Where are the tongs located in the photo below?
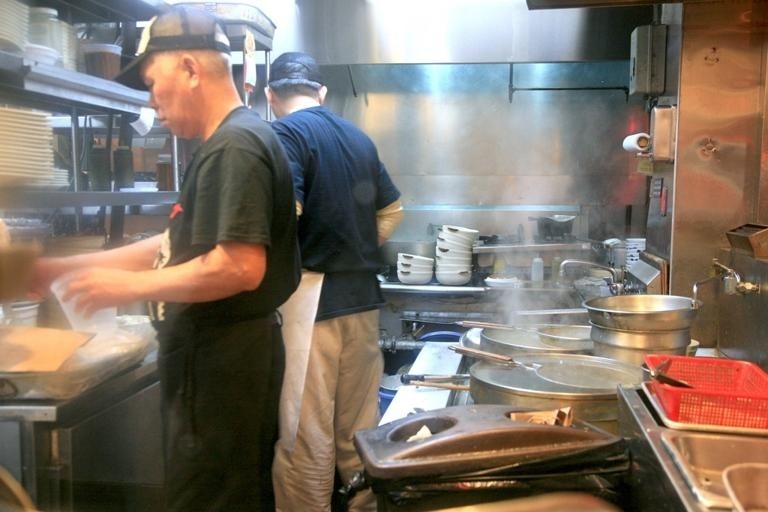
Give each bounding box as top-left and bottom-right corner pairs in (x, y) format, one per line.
(651, 358), (704, 388)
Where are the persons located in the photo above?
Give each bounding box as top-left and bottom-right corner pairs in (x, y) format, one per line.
(23, 7), (299, 512)
(263, 51), (405, 512)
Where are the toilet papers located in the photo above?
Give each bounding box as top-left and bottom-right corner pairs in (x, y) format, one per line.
(621, 132), (650, 153)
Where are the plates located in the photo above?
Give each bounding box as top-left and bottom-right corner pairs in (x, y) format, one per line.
(0, 0), (81, 71)
(0, 106), (72, 191)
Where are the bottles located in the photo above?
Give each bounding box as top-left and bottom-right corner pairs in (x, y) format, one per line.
(88, 143), (178, 193)
(531, 251), (563, 281)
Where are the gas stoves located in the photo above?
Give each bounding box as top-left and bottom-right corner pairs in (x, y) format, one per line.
(474, 232), (592, 252)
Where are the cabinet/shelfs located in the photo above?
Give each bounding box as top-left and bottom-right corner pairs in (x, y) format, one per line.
(0, 51), (192, 407)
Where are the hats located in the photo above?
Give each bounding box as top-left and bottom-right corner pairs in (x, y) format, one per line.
(267, 52), (322, 85)
(114, 9), (231, 91)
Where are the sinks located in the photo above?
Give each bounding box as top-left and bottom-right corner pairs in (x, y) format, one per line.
(507, 307), (591, 326)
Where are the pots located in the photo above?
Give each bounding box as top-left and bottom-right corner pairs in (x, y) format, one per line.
(530, 215), (577, 239)
(398, 309), (650, 436)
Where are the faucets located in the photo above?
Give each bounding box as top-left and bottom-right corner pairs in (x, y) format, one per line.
(691, 269), (725, 307)
(558, 259), (631, 297)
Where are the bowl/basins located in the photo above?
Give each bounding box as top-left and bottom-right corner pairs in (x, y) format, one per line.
(624, 236), (646, 272)
(394, 225), (480, 285)
(585, 293), (703, 332)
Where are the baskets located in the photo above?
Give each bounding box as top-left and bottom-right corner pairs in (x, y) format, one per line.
(644, 353), (768, 429)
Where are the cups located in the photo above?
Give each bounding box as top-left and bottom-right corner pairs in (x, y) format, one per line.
(48, 267), (117, 337)
(80, 42), (123, 82)
